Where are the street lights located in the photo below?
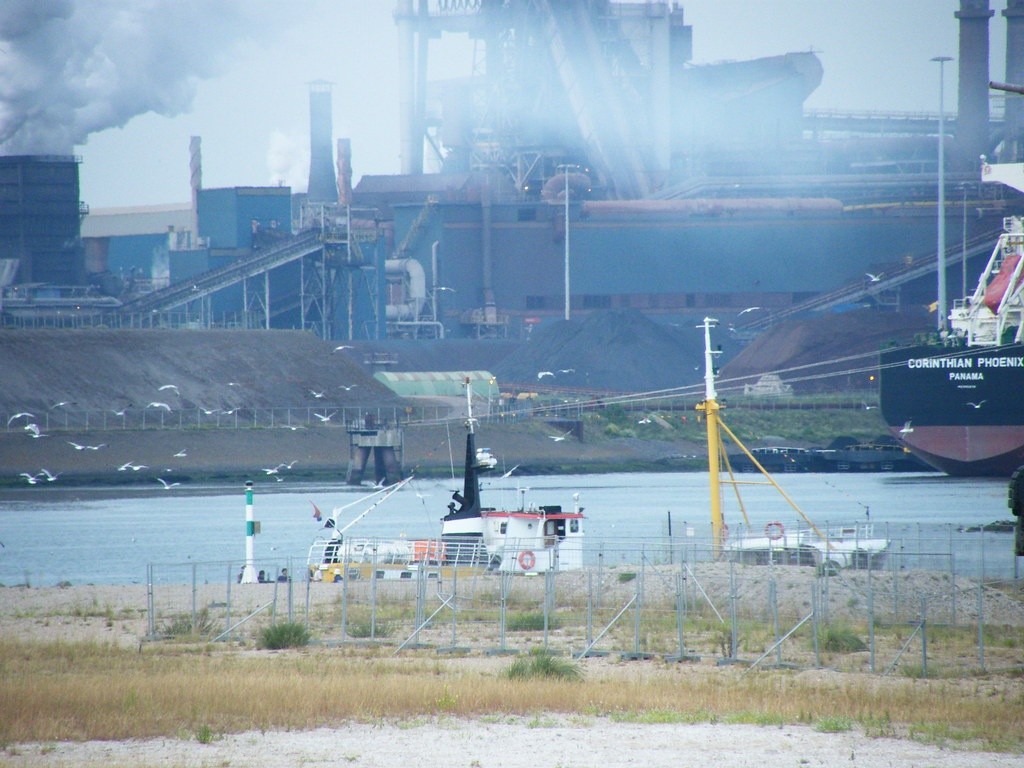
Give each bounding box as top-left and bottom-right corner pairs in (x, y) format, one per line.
(924, 55), (954, 331)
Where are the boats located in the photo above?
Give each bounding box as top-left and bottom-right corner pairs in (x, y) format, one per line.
(298, 373), (586, 577)
(878, 156), (1024, 483)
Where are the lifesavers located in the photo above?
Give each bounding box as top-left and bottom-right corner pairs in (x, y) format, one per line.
(821, 560), (841, 576)
(764, 520), (785, 541)
(851, 547), (868, 565)
(517, 550), (536, 570)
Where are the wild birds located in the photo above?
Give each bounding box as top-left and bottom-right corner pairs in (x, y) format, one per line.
(6, 273), (994, 500)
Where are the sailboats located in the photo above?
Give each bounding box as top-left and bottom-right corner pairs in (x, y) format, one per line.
(689, 314), (895, 572)
